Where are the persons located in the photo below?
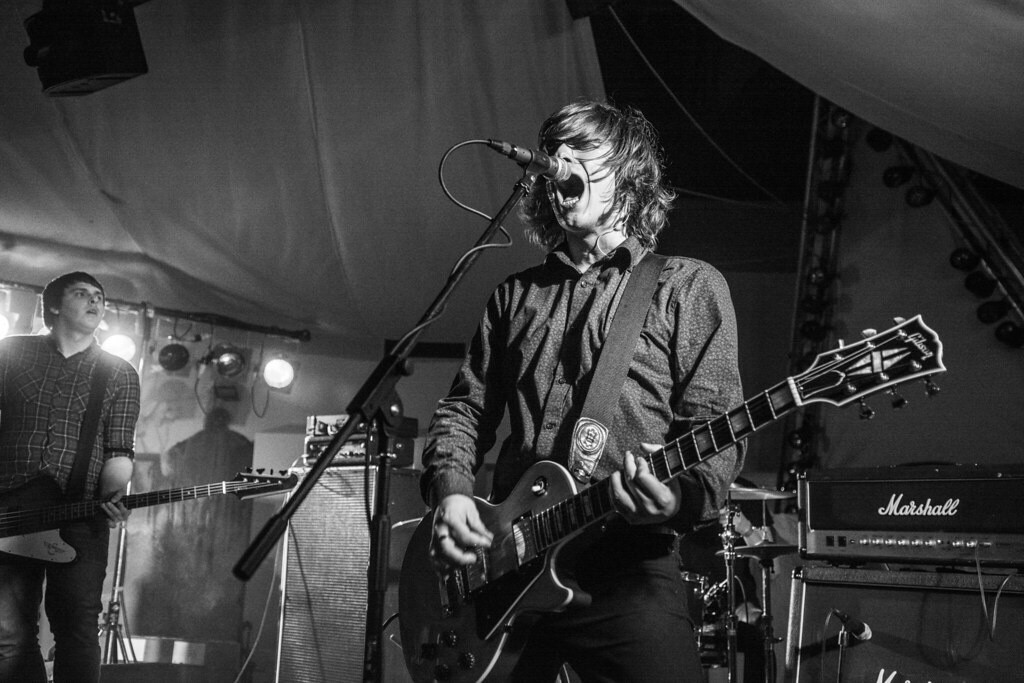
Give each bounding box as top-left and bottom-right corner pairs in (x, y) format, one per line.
(0, 269), (141, 683)
(418, 98), (749, 683)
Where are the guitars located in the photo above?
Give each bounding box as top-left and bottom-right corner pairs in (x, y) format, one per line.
(0, 465), (300, 566)
(400, 312), (949, 683)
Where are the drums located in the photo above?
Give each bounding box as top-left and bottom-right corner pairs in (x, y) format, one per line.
(676, 566), (730, 668)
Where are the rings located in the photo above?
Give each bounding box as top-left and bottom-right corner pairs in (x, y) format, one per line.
(435, 534), (451, 541)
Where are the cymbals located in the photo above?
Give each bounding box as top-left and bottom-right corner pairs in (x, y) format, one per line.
(714, 538), (810, 558)
(724, 479), (800, 501)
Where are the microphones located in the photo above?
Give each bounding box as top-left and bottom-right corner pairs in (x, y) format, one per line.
(834, 607), (872, 640)
(486, 138), (572, 184)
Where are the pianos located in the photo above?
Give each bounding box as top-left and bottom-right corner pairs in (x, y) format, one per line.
(797, 464), (1024, 566)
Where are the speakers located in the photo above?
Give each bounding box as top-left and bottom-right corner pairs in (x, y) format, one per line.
(271, 464), (431, 683)
(782, 565), (1024, 683)
